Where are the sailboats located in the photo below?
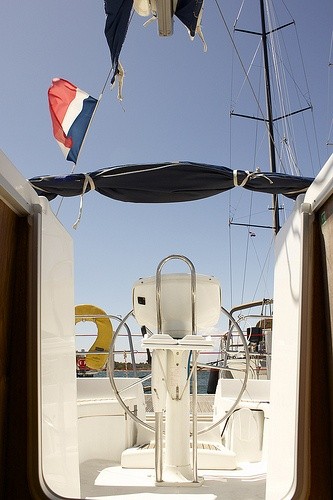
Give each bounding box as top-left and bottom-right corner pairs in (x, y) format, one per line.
(217, 1), (317, 380)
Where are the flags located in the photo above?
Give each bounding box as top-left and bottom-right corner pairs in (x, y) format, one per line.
(47, 76), (97, 167)
